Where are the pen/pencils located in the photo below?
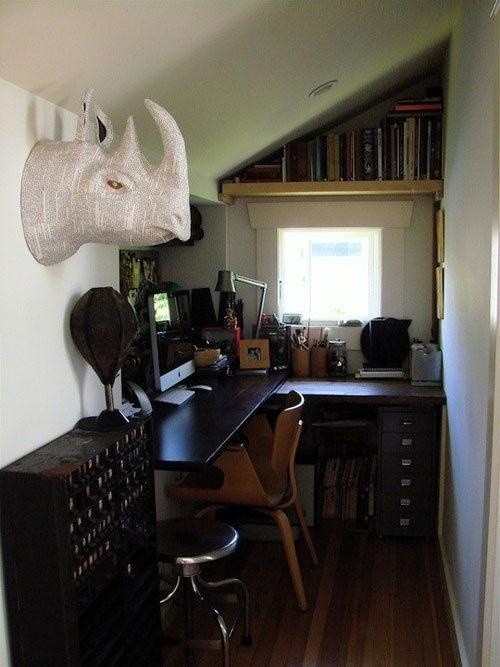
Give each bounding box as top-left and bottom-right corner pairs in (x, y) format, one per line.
(289, 327), (309, 350)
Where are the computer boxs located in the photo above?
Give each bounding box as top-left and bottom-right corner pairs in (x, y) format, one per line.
(221, 484), (301, 542)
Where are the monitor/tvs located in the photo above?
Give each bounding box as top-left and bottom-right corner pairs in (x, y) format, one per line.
(148, 290), (197, 392)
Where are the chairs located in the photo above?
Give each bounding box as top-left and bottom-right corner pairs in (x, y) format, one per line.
(165, 390), (319, 611)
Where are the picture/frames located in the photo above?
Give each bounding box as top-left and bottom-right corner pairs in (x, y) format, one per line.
(239, 340), (270, 369)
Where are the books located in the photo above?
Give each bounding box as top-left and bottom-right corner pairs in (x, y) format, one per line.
(352, 363), (405, 379)
(244, 95), (442, 183)
(322, 454), (377, 520)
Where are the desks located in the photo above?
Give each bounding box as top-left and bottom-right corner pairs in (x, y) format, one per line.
(146, 364), (285, 470)
(267, 374), (445, 404)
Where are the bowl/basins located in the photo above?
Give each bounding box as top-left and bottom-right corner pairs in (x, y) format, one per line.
(194, 349), (219, 366)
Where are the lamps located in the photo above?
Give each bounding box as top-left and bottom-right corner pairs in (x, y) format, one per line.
(213, 271), (267, 339)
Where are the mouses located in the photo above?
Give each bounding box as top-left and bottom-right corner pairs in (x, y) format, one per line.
(191, 385), (212, 391)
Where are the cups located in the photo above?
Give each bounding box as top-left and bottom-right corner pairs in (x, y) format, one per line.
(290, 348), (310, 377)
(310, 346), (328, 377)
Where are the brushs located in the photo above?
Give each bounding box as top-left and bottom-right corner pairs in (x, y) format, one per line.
(323, 328), (330, 343)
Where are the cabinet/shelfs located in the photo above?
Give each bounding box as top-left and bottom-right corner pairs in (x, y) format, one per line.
(374, 406), (438, 540)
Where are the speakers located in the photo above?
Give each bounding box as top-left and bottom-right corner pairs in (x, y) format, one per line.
(125, 379), (154, 415)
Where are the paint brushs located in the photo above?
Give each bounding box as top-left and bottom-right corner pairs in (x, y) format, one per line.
(325, 326), (328, 342)
(320, 327), (323, 342)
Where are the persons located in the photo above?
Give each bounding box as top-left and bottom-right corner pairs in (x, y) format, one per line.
(248, 348), (261, 360)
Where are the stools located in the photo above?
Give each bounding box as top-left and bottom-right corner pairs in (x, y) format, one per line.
(156, 517), (252, 667)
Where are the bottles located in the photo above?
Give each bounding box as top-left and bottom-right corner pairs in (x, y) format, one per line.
(326, 341), (347, 378)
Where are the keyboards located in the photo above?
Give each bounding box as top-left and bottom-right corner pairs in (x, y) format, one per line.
(152, 387), (195, 407)
